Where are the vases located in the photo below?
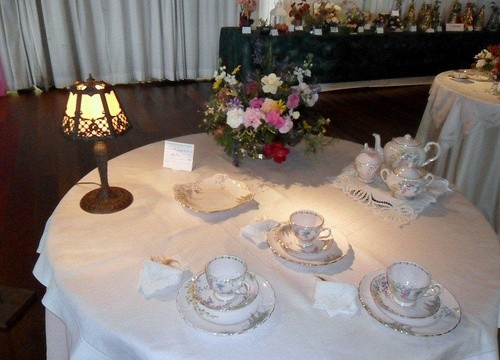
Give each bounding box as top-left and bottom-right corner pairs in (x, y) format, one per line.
(240, 22), (250, 31)
(356, 24), (363, 33)
(493, 71), (500, 81)
(321, 20), (329, 33)
(249, 129), (285, 159)
(294, 20), (303, 31)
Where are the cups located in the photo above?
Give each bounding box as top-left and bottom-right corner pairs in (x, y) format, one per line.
(205, 254), (250, 296)
(386, 261), (443, 309)
(289, 209), (332, 247)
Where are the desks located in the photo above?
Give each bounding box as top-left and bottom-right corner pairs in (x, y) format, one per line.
(35, 128), (500, 360)
(413, 68), (500, 249)
(220, 26), (498, 84)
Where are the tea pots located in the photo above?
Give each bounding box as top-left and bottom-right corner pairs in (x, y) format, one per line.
(371, 133), (442, 171)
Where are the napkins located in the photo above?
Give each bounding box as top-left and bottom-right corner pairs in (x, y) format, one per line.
(313, 277), (362, 321)
(240, 218), (283, 247)
(136, 255), (189, 297)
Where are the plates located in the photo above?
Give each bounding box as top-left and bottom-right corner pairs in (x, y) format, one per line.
(370, 273), (441, 319)
(266, 221), (350, 266)
(273, 220), (335, 255)
(357, 269), (462, 338)
(177, 271), (276, 336)
(171, 172), (254, 215)
(191, 270), (259, 310)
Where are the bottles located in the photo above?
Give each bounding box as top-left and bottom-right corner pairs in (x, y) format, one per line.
(379, 161), (434, 200)
(354, 143), (380, 183)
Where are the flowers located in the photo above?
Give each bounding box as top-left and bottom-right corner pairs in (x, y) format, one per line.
(472, 44), (500, 70)
(375, 12), (402, 26)
(312, 1), (340, 20)
(344, 8), (372, 26)
(237, 0), (258, 26)
(193, 51), (331, 164)
(287, 1), (312, 20)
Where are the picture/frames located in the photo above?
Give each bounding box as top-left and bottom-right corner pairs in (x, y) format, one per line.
(383, 26), (390, 32)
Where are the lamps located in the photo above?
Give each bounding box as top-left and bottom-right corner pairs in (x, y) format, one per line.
(61, 74), (137, 216)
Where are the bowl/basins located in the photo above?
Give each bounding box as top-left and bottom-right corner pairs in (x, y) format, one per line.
(454, 70), (467, 79)
